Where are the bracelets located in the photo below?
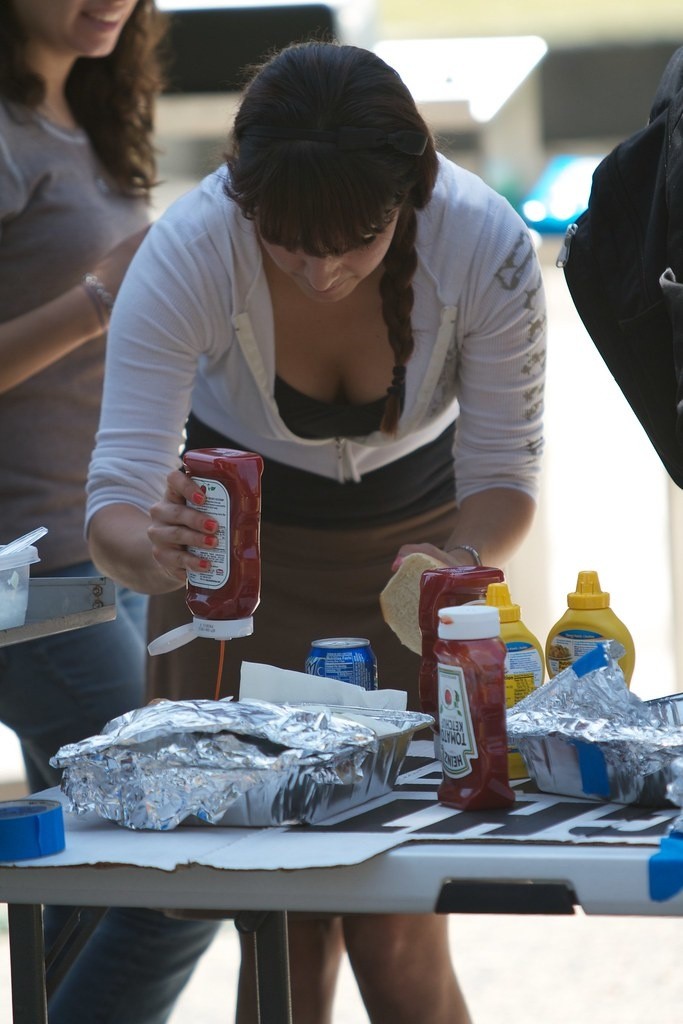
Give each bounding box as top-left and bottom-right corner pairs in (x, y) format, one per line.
(81, 272), (115, 331)
(446, 542), (483, 566)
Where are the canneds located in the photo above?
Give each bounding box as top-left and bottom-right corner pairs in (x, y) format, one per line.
(304, 637), (378, 691)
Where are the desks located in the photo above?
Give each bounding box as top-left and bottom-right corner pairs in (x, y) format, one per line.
(0, 739), (683, 1024)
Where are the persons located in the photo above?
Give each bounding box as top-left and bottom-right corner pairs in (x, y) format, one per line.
(81, 42), (550, 1024)
(0, 0), (225, 1024)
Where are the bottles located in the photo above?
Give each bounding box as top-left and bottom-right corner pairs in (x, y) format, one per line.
(473, 582), (546, 779)
(435, 605), (515, 810)
(545, 570), (635, 693)
(418, 564), (505, 764)
(178, 446), (263, 640)
